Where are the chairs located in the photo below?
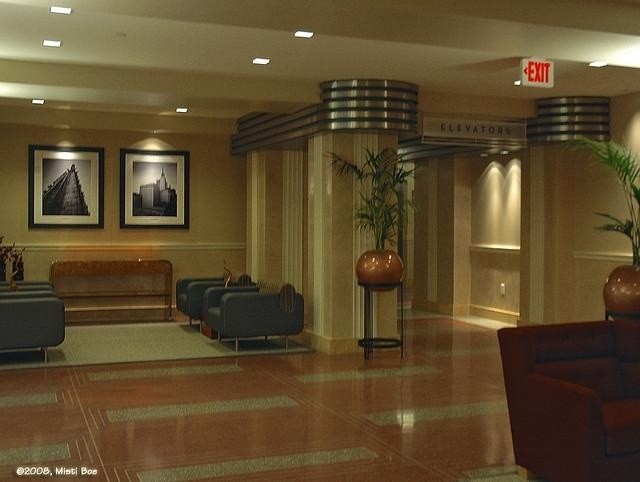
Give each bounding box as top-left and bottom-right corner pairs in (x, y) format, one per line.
(0, 279), (54, 291)
(202, 279), (305, 352)
(0, 290), (66, 362)
(176, 268), (251, 330)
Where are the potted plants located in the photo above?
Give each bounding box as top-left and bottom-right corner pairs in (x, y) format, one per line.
(325, 146), (418, 288)
(568, 132), (639, 319)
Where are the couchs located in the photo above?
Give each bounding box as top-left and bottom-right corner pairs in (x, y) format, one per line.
(497, 320), (640, 482)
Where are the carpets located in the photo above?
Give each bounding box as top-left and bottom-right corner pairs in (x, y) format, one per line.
(0, 319), (316, 371)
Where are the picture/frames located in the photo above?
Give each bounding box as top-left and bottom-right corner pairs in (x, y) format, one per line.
(120, 148), (192, 230)
(27, 144), (105, 230)
